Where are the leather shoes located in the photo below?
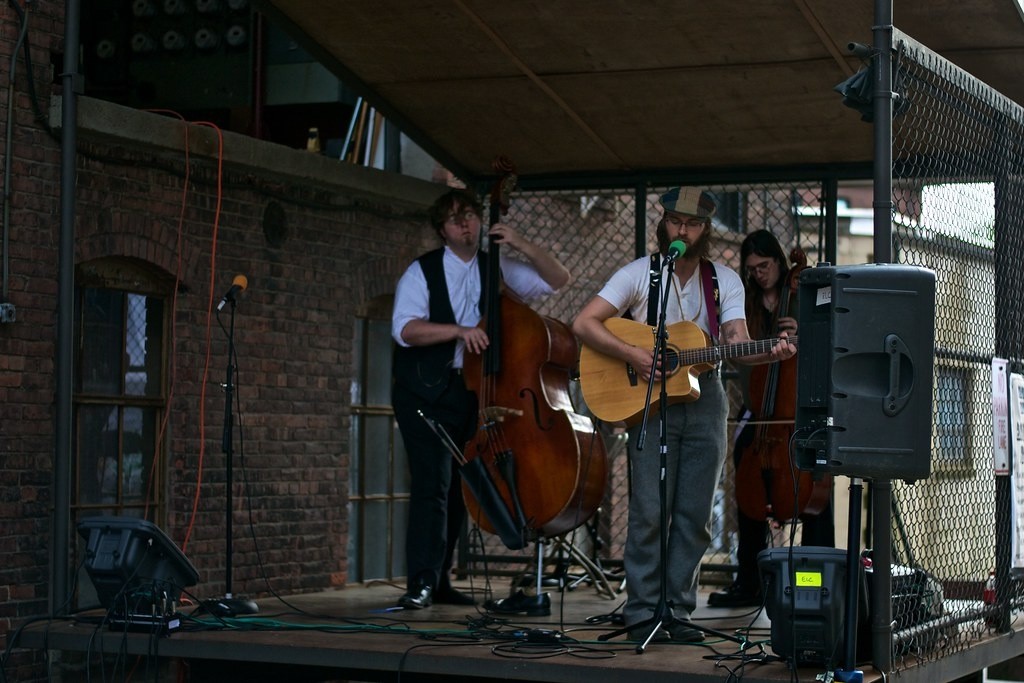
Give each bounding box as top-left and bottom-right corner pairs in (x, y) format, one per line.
(398, 584), (432, 610)
(483, 589), (550, 617)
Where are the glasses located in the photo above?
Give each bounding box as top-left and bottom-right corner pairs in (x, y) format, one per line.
(746, 263), (775, 274)
(665, 213), (706, 231)
(445, 210), (479, 225)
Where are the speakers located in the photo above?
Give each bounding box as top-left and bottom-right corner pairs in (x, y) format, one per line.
(793, 262), (935, 484)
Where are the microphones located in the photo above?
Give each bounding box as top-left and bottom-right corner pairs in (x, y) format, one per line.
(217, 275), (248, 312)
(662, 240), (686, 266)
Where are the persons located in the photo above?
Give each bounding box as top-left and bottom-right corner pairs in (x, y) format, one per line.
(706, 230), (838, 610)
(390, 190), (570, 610)
(573, 187), (797, 644)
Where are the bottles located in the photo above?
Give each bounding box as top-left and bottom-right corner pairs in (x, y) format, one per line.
(984, 572), (997, 627)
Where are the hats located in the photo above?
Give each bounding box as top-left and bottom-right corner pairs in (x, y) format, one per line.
(659, 185), (717, 222)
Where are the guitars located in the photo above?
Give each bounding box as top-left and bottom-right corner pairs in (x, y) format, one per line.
(577, 313), (798, 430)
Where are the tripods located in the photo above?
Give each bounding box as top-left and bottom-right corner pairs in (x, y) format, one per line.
(598, 255), (750, 653)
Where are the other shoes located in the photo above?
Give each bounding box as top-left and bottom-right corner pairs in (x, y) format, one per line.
(665, 619), (706, 642)
(626, 622), (670, 642)
(709, 586), (762, 608)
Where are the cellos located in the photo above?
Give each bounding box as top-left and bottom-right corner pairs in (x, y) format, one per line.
(733, 244), (834, 522)
(458, 153), (613, 597)
